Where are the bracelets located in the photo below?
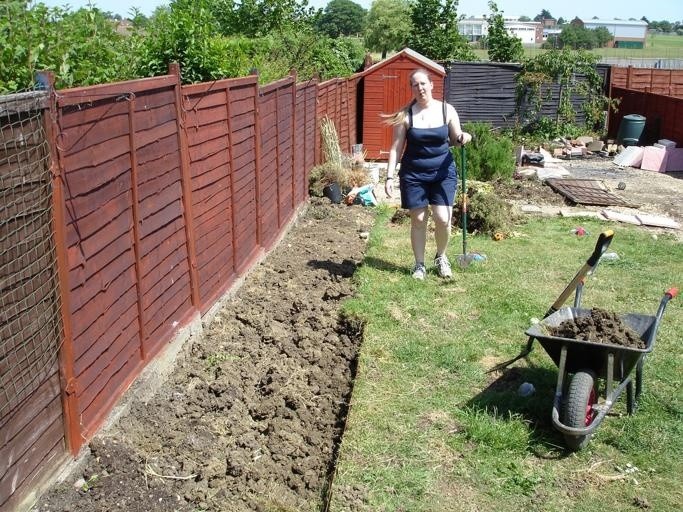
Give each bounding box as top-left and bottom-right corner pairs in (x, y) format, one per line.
(387, 177), (394, 181)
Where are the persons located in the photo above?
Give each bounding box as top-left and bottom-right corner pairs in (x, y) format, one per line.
(384, 68), (472, 280)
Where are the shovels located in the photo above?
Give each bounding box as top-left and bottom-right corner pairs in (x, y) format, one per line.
(454, 139), (486, 267)
(486, 229), (615, 374)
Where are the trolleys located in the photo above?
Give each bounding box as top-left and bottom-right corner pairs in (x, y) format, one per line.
(524, 252), (680, 451)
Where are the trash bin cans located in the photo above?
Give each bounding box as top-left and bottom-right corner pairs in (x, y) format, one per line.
(617, 114), (647, 145)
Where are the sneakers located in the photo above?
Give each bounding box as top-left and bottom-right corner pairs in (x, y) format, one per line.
(433, 251), (453, 278)
(410, 264), (427, 282)
